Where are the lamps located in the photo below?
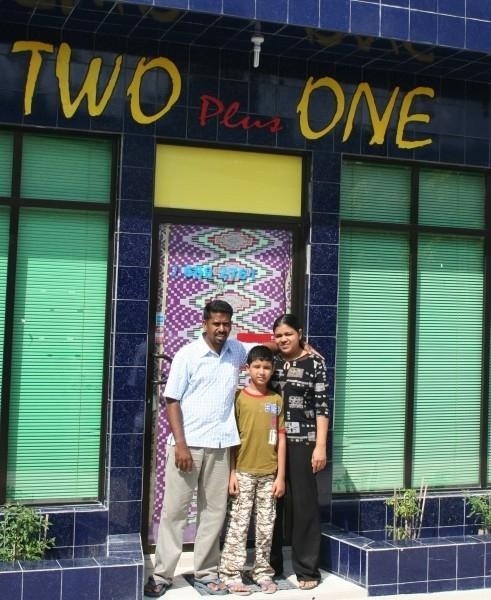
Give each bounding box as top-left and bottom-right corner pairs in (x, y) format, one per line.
(250, 32), (264, 70)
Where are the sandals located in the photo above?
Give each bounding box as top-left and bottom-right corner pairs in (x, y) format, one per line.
(194, 578), (228, 595)
(299, 579), (320, 590)
(254, 578), (277, 594)
(145, 578), (174, 599)
(223, 581), (252, 597)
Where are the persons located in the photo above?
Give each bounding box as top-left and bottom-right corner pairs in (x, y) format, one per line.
(268, 315), (330, 590)
(218, 347), (286, 596)
(144, 300), (326, 596)
